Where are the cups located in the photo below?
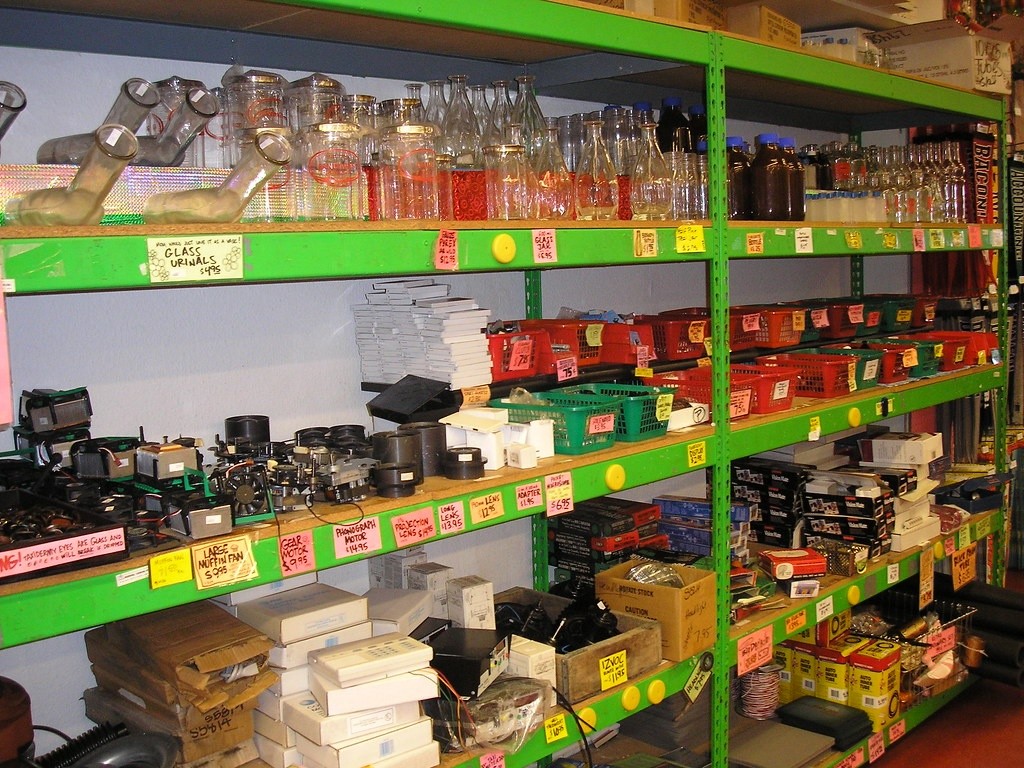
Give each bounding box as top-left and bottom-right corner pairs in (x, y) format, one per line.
(158, 67), (452, 225)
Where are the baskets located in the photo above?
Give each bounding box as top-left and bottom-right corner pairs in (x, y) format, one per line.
(476, 291), (944, 382)
(486, 392), (626, 456)
(633, 328), (1001, 421)
(549, 384), (678, 443)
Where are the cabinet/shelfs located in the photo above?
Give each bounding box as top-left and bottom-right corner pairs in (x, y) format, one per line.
(0, 0), (1024, 768)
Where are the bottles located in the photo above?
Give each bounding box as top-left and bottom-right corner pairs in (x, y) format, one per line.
(803, 38), (855, 60)
(751, 133), (805, 220)
(883, 142), (968, 223)
(726, 136), (751, 220)
(405, 74), (708, 221)
(804, 141), (883, 192)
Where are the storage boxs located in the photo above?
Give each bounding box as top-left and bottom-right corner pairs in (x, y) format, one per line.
(585, 0), (1024, 96)
(79, 375), (1002, 768)
